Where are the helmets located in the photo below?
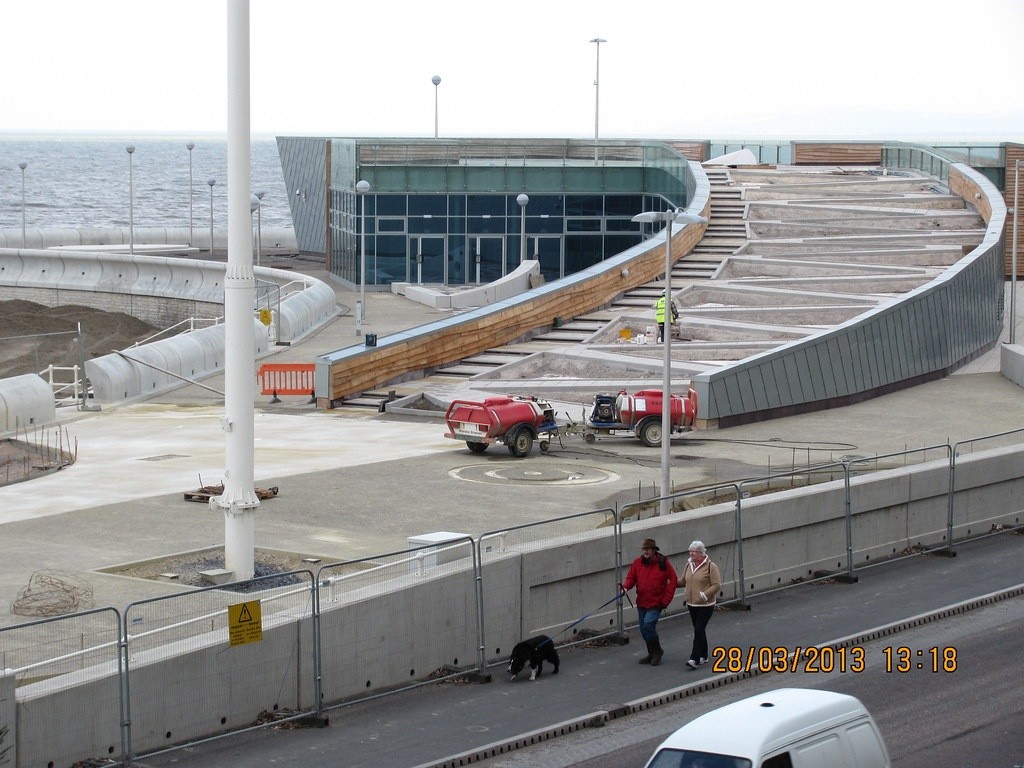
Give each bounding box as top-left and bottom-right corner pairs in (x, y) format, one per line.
(662, 288), (666, 296)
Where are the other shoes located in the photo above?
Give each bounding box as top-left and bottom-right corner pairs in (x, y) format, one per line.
(640, 655), (652, 664)
(651, 650), (663, 665)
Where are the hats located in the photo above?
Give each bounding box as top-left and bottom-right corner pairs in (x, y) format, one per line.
(640, 539), (660, 551)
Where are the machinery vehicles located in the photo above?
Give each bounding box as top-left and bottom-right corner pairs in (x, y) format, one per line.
(443, 393), (559, 458)
(587, 388), (697, 447)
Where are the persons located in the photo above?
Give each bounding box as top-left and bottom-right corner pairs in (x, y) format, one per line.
(620, 539), (677, 666)
(668, 541), (721, 669)
(656, 289), (679, 342)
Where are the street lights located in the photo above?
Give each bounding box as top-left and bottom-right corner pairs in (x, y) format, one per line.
(589, 38), (608, 165)
(19, 161), (28, 246)
(254, 190), (264, 267)
(126, 143), (135, 254)
(631, 210), (704, 516)
(356, 179), (370, 320)
(208, 178), (215, 254)
(432, 75), (441, 137)
(186, 142), (194, 246)
(517, 193), (529, 264)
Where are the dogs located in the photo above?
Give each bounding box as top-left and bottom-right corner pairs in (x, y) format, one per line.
(507, 634), (560, 681)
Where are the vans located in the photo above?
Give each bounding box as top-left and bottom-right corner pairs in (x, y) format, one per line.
(644, 688), (892, 768)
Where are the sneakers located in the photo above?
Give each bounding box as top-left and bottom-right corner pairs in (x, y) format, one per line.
(699, 657), (709, 664)
(686, 660), (698, 668)
